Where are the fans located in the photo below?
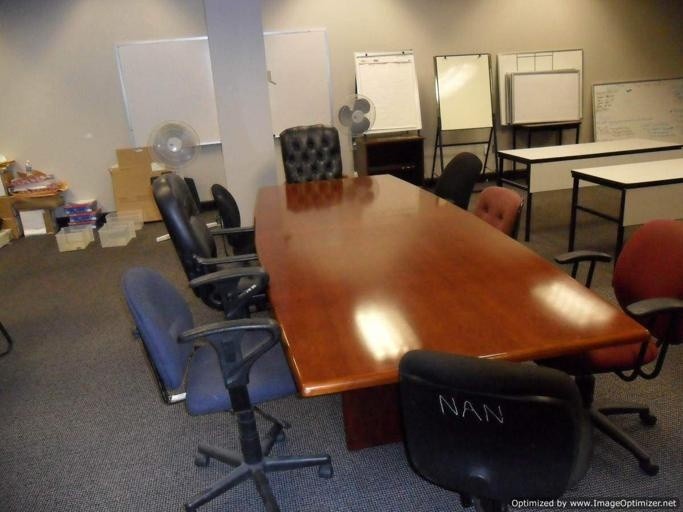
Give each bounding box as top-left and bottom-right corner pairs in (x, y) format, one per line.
(147, 120), (222, 242)
(335, 93), (376, 177)
(345, 177), (378, 208)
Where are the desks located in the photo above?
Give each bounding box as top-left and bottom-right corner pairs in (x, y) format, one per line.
(513, 121), (582, 179)
(498, 137), (682, 242)
(566, 157), (683, 287)
(256, 174), (650, 453)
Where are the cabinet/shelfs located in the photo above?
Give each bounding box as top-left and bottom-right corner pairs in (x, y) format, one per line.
(356, 135), (427, 189)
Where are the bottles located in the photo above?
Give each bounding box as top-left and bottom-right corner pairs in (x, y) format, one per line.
(25, 160), (32, 176)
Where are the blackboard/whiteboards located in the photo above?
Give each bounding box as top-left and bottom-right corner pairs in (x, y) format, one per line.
(592, 77), (683, 142)
(354, 51), (421, 137)
(495, 49), (584, 127)
(433, 53), (492, 131)
(511, 71), (581, 123)
(115, 29), (335, 151)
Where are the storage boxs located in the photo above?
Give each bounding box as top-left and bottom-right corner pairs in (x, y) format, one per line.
(54, 200), (102, 253)
(0, 195), (65, 249)
(97, 145), (166, 248)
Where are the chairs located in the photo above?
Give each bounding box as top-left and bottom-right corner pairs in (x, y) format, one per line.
(285, 180), (343, 211)
(399, 349), (588, 512)
(121, 265), (334, 512)
(435, 152), (483, 210)
(279, 124), (343, 182)
(534, 219), (683, 475)
(153, 173), (271, 317)
(473, 186), (525, 241)
